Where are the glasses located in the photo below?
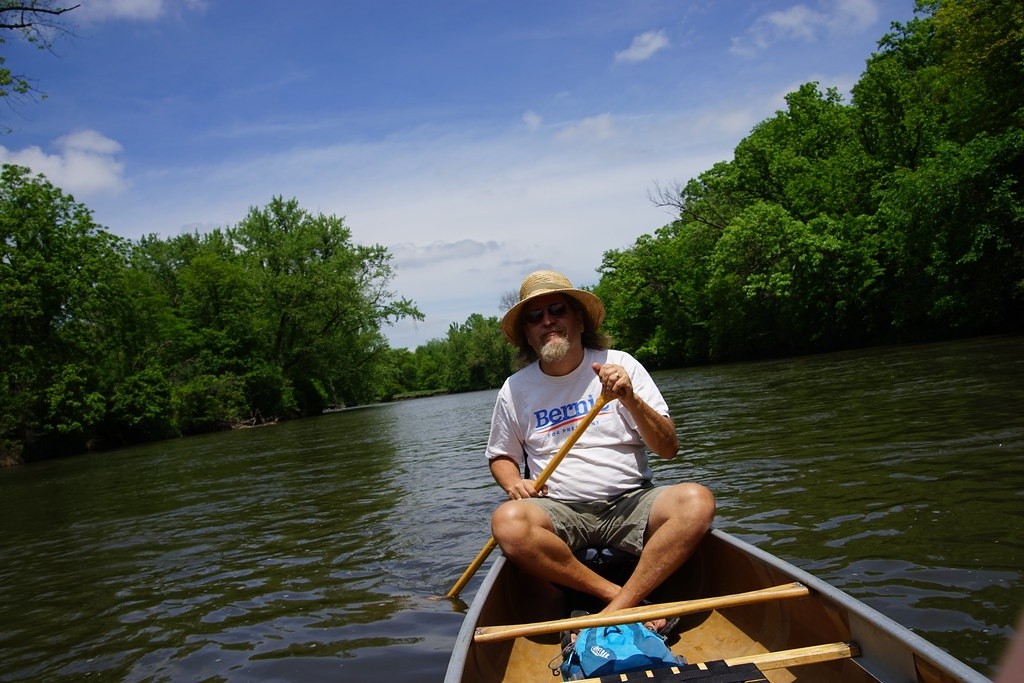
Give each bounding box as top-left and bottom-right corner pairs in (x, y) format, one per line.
(522, 303), (572, 325)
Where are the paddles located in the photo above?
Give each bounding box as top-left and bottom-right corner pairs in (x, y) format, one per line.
(446, 393), (618, 598)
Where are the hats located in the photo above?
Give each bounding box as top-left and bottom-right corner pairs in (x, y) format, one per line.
(501, 270), (605, 348)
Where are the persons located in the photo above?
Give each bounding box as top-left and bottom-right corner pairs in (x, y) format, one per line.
(485, 270), (715, 657)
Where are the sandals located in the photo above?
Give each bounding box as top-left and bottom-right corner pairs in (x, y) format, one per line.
(643, 616), (680, 640)
(560, 610), (590, 660)
